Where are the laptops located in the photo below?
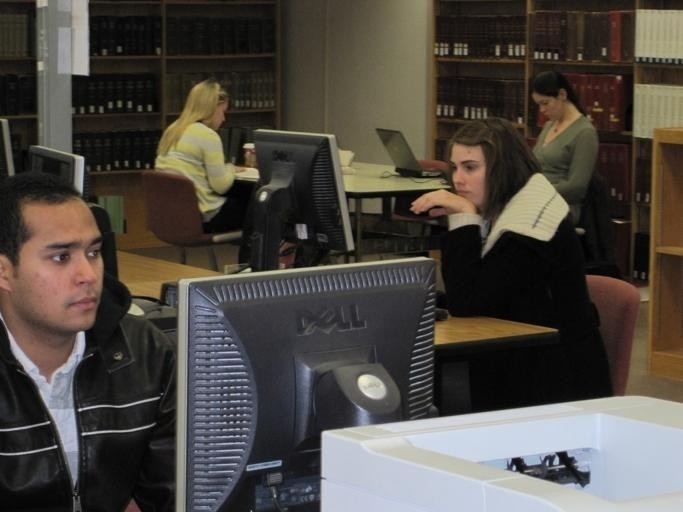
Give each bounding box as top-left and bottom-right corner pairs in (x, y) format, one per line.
(375, 128), (444, 178)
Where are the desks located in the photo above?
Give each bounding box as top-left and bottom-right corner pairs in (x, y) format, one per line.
(117, 251), (563, 416)
(231, 160), (451, 260)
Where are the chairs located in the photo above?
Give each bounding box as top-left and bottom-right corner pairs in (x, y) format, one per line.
(584, 273), (639, 395)
(142, 170), (246, 271)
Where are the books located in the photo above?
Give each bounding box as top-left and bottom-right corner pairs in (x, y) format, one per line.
(1, 1), (277, 60)
(434, 1), (680, 284)
(0, 71), (276, 172)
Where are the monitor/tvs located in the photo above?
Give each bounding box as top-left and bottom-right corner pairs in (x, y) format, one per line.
(27, 144), (90, 203)
(0, 118), (16, 178)
(174, 255), (437, 511)
(253, 128), (355, 253)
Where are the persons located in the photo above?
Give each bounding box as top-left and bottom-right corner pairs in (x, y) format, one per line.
(152, 76), (257, 268)
(410, 118), (614, 414)
(0, 171), (176, 512)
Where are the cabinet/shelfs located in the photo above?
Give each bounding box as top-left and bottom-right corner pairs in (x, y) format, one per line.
(423, 0), (683, 383)
(0, 0), (284, 249)
